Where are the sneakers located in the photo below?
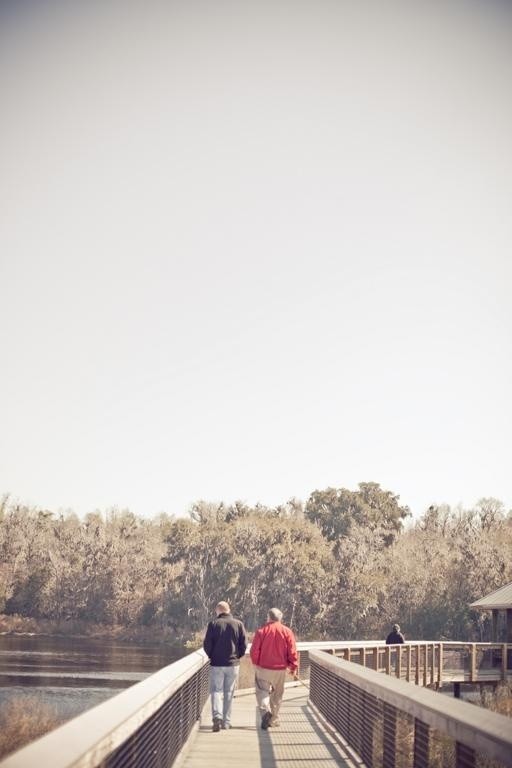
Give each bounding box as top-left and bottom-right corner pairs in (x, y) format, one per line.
(212, 711), (280, 732)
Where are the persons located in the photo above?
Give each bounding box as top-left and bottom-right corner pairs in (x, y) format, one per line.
(250, 606), (300, 730)
(386, 623), (405, 644)
(203, 600), (248, 732)
